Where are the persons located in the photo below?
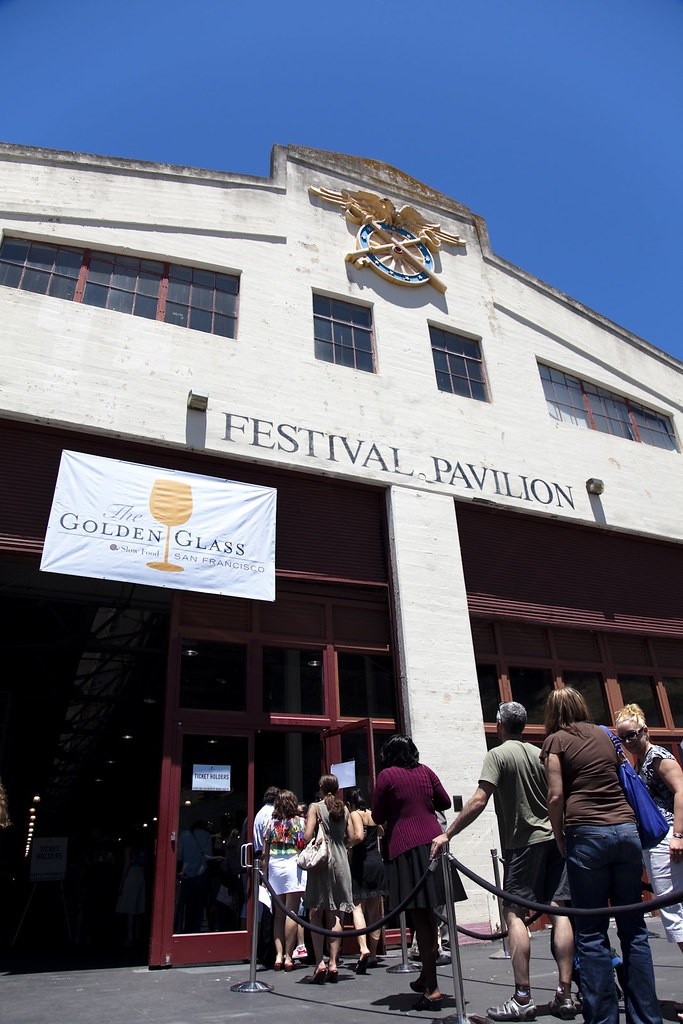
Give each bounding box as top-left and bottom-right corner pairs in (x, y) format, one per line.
(253, 774), (451, 982)
(371, 734), (451, 1011)
(430, 687), (683, 1024)
(114, 801), (247, 945)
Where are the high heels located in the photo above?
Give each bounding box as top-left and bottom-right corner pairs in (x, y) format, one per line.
(327, 969), (338, 983)
(412, 994), (441, 1011)
(309, 967), (327, 985)
(410, 975), (427, 993)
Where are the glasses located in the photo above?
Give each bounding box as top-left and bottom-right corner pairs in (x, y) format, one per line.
(618, 726), (644, 742)
(497, 701), (508, 714)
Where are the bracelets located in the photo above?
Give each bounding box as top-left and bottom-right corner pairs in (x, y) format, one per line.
(673, 833), (683, 838)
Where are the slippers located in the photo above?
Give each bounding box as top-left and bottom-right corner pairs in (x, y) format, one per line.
(356, 953), (372, 975)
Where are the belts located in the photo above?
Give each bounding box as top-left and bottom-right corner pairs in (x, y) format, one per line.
(255, 850), (262, 857)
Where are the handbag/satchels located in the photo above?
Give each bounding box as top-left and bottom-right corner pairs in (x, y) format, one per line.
(600, 725), (670, 850)
(196, 867), (214, 897)
(296, 803), (329, 871)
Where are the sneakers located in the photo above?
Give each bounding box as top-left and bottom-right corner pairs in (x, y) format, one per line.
(548, 994), (576, 1016)
(486, 995), (537, 1021)
(283, 947), (308, 958)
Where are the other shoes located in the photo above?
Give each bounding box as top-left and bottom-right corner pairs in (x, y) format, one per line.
(327, 958), (339, 966)
(436, 955), (452, 965)
(410, 954), (422, 961)
(284, 963), (295, 972)
(274, 962), (284, 971)
(366, 956), (377, 968)
